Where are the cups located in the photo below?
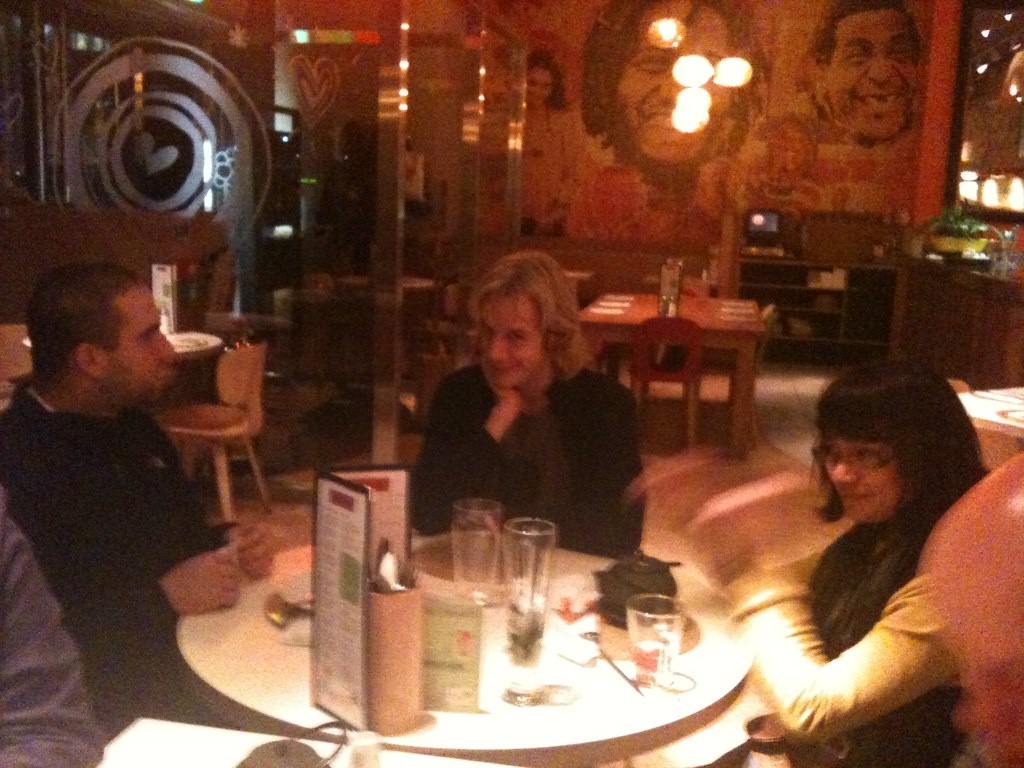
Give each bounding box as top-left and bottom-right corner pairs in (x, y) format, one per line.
(625, 593), (686, 686)
(450, 498), (505, 604)
(503, 517), (558, 631)
(366, 587), (425, 733)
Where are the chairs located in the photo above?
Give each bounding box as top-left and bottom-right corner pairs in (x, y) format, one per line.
(630, 316), (705, 446)
(157, 342), (273, 526)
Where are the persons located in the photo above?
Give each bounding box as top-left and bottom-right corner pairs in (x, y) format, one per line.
(0, 258), (282, 768)
(405, 251), (646, 560)
(695, 365), (1024, 768)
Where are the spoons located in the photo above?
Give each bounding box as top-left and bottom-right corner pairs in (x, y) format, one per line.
(582, 631), (643, 695)
(377, 550), (399, 593)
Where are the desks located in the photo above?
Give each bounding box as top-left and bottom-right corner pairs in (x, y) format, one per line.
(578, 291), (767, 460)
(175, 533), (754, 768)
(98, 717), (522, 768)
(164, 332), (225, 360)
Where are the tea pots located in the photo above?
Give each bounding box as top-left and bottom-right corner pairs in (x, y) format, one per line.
(595, 549), (683, 613)
(234, 721), (346, 768)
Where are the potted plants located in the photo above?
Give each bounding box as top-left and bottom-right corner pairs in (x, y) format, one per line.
(987, 225), (1018, 279)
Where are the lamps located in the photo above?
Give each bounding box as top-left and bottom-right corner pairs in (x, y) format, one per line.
(648, 0), (754, 134)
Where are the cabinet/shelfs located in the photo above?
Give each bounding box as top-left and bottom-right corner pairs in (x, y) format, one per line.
(732, 256), (895, 348)
(927, 198), (990, 253)
